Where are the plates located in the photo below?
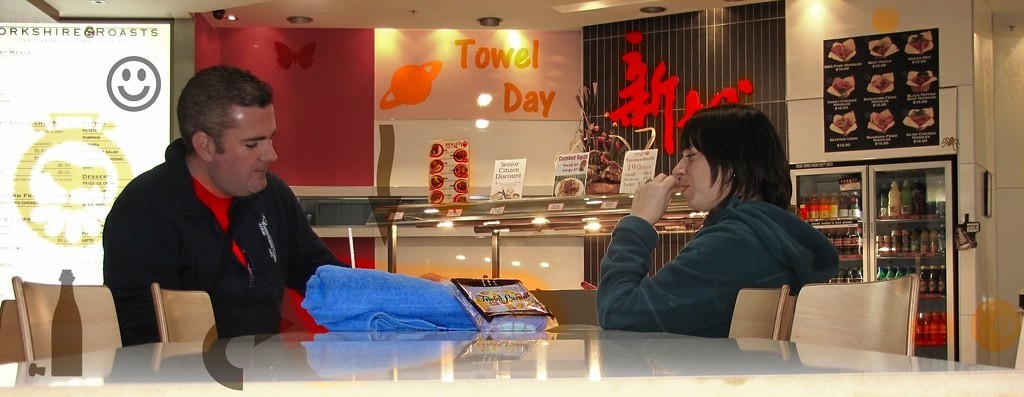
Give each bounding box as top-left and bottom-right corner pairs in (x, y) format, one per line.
(554, 178), (585, 198)
(454, 179), (468, 194)
(430, 159), (444, 173)
(453, 149), (468, 163)
(429, 144), (444, 157)
(453, 193), (466, 203)
(430, 175), (444, 190)
(429, 189), (444, 204)
(453, 164), (469, 178)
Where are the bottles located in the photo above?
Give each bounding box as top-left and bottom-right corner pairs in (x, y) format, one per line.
(798, 175), (948, 346)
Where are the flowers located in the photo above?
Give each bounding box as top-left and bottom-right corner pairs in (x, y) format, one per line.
(567, 83), (626, 183)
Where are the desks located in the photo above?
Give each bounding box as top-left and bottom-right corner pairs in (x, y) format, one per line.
(1, 326), (1015, 389)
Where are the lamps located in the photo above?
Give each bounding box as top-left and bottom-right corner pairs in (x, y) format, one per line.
(479, 18), (500, 27)
(290, 17), (308, 24)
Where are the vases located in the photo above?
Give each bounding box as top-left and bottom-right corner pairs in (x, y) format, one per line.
(587, 183), (619, 195)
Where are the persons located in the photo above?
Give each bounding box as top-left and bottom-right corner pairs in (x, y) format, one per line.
(102, 65), (352, 347)
(595, 103), (839, 338)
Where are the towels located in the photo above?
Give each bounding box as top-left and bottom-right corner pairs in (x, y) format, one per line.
(301, 264), (480, 333)
(297, 331), (469, 380)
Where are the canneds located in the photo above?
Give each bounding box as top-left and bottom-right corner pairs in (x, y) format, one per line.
(875, 228), (910, 257)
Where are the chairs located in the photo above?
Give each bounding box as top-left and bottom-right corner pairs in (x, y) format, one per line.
(729, 282), (790, 341)
(788, 272), (921, 357)
(0, 299), (25, 366)
(152, 282), (218, 343)
(11, 276), (123, 363)
